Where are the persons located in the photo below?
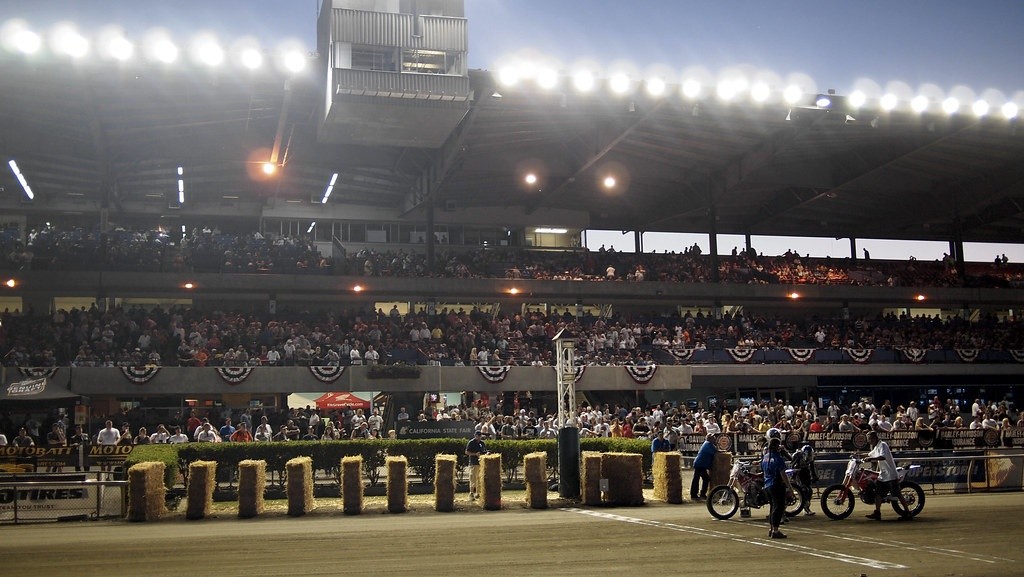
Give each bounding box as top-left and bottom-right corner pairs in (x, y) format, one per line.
(825, 395), (1024, 454)
(0, 223), (336, 273)
(717, 247), (1024, 290)
(690, 433), (717, 504)
(389, 393), (558, 440)
(465, 430), (489, 501)
(562, 396), (825, 471)
(0, 302), (466, 367)
(753, 428), (822, 539)
(853, 430), (913, 521)
(0, 403), (273, 471)
(467, 306), (802, 367)
(273, 405), (384, 442)
(352, 233), (710, 283)
(806, 310), (1024, 350)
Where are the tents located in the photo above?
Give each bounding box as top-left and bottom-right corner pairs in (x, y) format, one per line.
(0, 376), (92, 446)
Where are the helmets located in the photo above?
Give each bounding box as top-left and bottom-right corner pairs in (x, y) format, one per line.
(802, 444), (814, 461)
(767, 427), (780, 438)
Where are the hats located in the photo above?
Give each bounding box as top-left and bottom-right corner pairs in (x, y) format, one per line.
(19, 427), (26, 432)
(122, 421), (130, 428)
(666, 417), (673, 421)
(708, 413), (715, 417)
(898, 414), (903, 417)
(706, 433), (715, 439)
(520, 409), (526, 413)
(474, 430), (481, 435)
(280, 424), (287, 429)
(909, 400), (917, 406)
(658, 430), (663, 435)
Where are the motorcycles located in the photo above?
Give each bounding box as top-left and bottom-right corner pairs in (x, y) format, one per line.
(706, 460), (806, 519)
(821, 453), (925, 519)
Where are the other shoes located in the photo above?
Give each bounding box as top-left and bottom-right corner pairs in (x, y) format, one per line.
(768, 528), (787, 539)
(897, 512), (913, 520)
(691, 495), (707, 500)
(806, 511), (815, 515)
(866, 511), (882, 521)
